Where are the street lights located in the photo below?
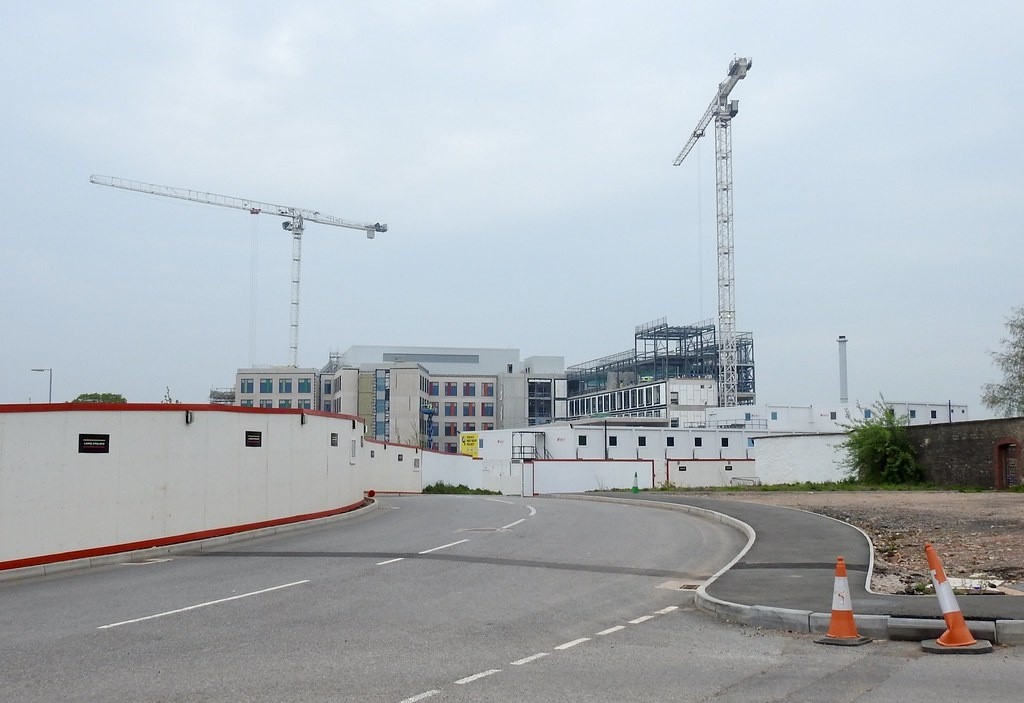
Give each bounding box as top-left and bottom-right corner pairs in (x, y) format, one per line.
(30, 368), (53, 404)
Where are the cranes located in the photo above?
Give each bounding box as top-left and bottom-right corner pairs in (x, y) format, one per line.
(671, 54), (756, 408)
(87, 174), (387, 366)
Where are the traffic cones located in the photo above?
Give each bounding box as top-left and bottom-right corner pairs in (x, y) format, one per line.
(812, 556), (874, 646)
(920, 543), (995, 654)
